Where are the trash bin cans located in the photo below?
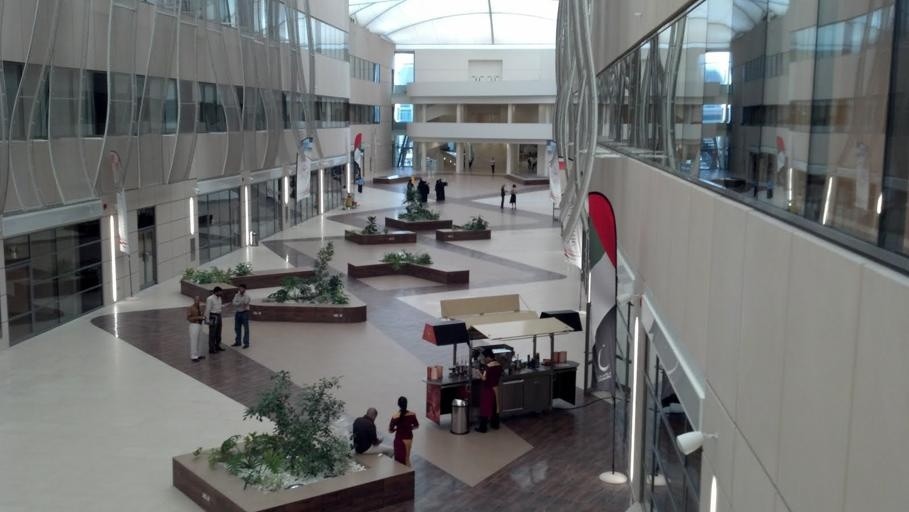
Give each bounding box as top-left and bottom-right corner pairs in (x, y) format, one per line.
(250, 231), (258, 246)
(451, 399), (469, 434)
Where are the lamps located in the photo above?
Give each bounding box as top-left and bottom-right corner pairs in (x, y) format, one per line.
(617, 293), (642, 306)
(676, 431), (719, 456)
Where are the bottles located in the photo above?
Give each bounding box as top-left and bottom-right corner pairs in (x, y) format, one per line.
(456, 361), (468, 377)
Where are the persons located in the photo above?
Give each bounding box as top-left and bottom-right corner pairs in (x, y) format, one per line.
(509, 184), (517, 211)
(231, 284), (251, 348)
(526, 151), (538, 172)
(204, 286), (225, 353)
(403, 175), (447, 207)
(489, 157), (496, 175)
(345, 193), (357, 209)
(500, 184), (508, 209)
(474, 349), (504, 433)
(357, 174), (362, 193)
(352, 408), (394, 459)
(388, 395), (420, 467)
(341, 185), (348, 210)
(185, 296), (205, 361)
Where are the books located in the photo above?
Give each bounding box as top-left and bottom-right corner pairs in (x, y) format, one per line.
(206, 315), (218, 324)
